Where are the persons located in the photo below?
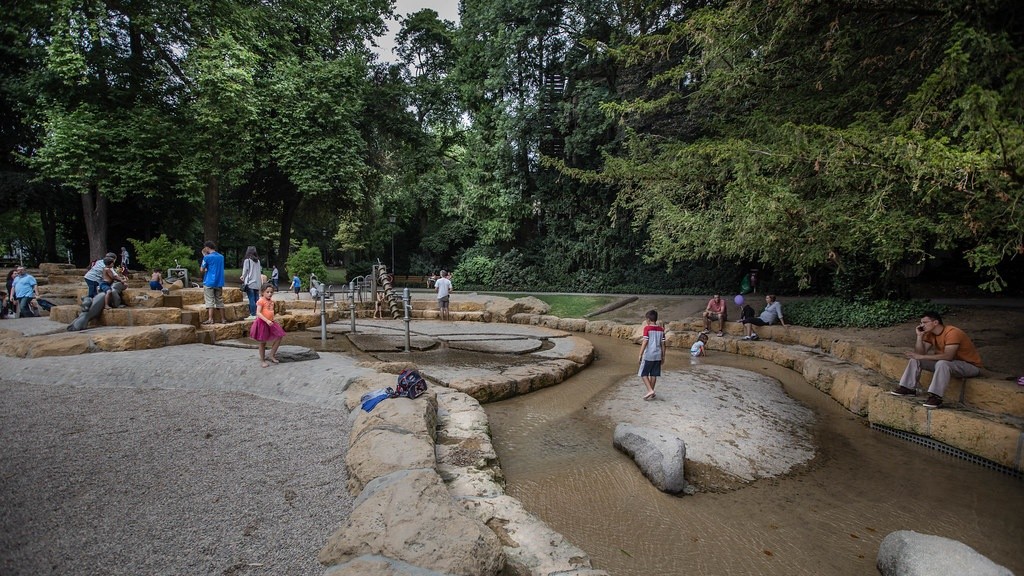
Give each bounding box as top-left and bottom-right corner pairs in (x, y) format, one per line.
(691, 333), (708, 357)
(239, 246), (268, 321)
(80, 252), (129, 310)
(427, 272), (453, 289)
(637, 310), (666, 401)
(120, 247), (129, 281)
(373, 291), (384, 319)
(250, 283), (286, 368)
(699, 293), (727, 337)
(735, 294), (791, 341)
(200, 240), (226, 324)
(149, 267), (163, 290)
(434, 270), (453, 321)
(289, 273), (301, 301)
(0, 266), (57, 319)
(890, 311), (983, 407)
(271, 265), (279, 292)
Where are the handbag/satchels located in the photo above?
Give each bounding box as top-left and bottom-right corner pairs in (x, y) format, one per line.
(240, 284), (249, 291)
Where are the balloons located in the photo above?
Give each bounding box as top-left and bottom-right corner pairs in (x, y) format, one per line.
(734, 295), (744, 306)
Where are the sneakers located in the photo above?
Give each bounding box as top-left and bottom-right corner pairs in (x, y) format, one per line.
(922, 396), (943, 408)
(890, 386), (916, 397)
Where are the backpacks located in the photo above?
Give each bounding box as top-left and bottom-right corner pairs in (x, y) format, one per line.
(395, 369), (427, 399)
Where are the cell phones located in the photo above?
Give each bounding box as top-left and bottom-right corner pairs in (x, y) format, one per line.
(919, 327), (924, 331)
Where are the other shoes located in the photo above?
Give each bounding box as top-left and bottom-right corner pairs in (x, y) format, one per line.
(702, 329), (710, 334)
(743, 335), (758, 340)
(219, 319), (226, 324)
(717, 331), (723, 337)
(244, 315), (257, 320)
(203, 319), (214, 324)
